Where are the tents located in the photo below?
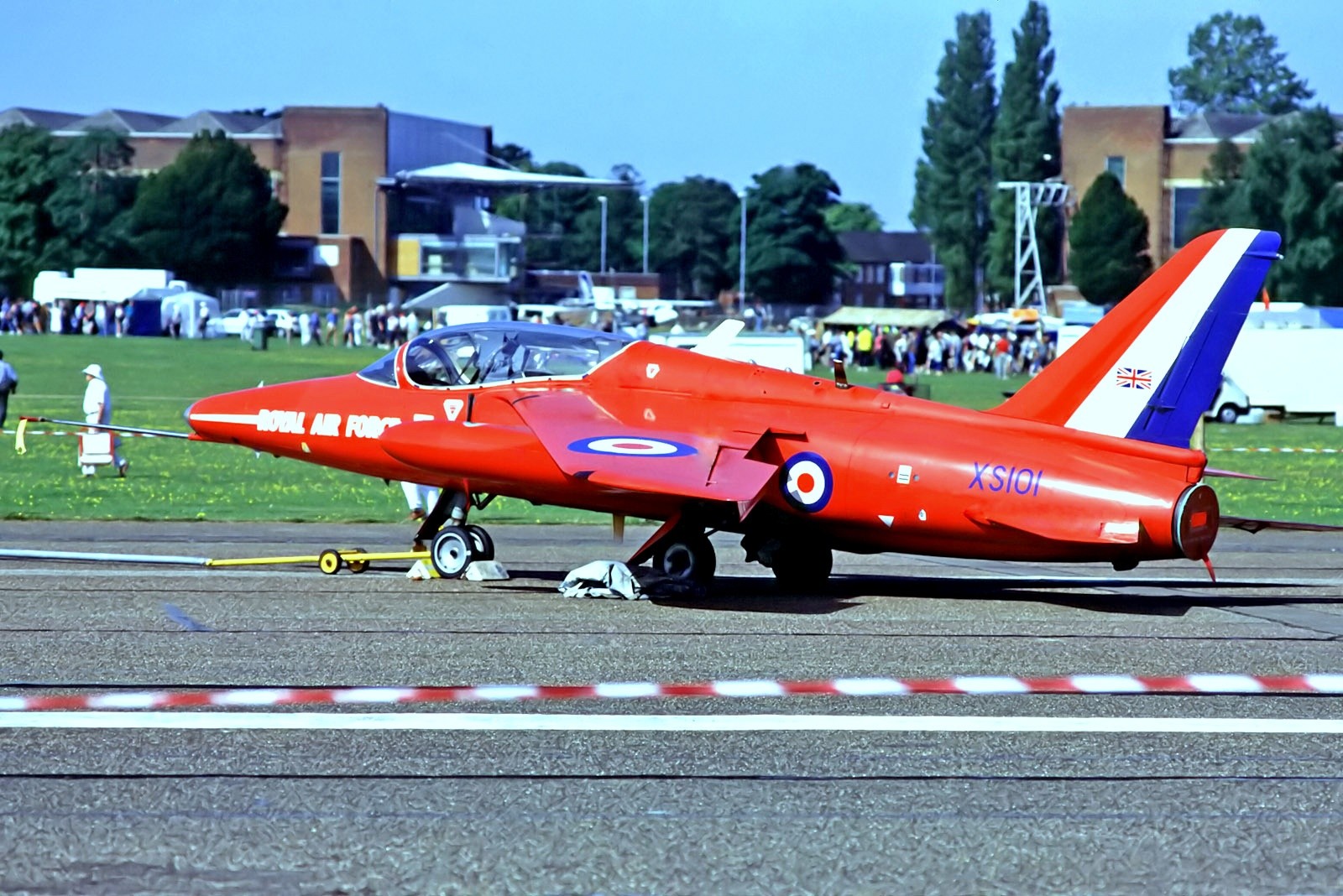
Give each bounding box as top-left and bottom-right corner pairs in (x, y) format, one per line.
(821, 306), (967, 330)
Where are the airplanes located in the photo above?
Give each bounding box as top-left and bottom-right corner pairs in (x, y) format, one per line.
(17, 225), (1286, 601)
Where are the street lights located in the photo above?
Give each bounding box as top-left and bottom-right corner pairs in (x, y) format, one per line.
(597, 195), (607, 270)
(638, 195), (651, 273)
(735, 191), (749, 316)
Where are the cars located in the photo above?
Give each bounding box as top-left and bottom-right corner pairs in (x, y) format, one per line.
(1205, 372), (1251, 426)
(264, 308), (301, 339)
(205, 307), (275, 337)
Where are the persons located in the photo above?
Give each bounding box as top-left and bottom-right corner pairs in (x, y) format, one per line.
(0, 349), (18, 429)
(636, 317), (650, 342)
(741, 296), (1057, 381)
(401, 481), (439, 522)
(537, 312), (564, 326)
(80, 364), (130, 479)
(612, 303), (627, 332)
(0, 280), (435, 353)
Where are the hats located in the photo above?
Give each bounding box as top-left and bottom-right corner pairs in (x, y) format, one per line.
(82, 364), (104, 380)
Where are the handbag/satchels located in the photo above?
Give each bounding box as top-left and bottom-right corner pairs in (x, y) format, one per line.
(79, 433), (113, 465)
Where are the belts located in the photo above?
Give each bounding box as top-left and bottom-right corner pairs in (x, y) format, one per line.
(86, 411), (98, 416)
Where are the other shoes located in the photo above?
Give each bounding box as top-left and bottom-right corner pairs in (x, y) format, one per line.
(120, 463), (128, 478)
(82, 474), (95, 478)
